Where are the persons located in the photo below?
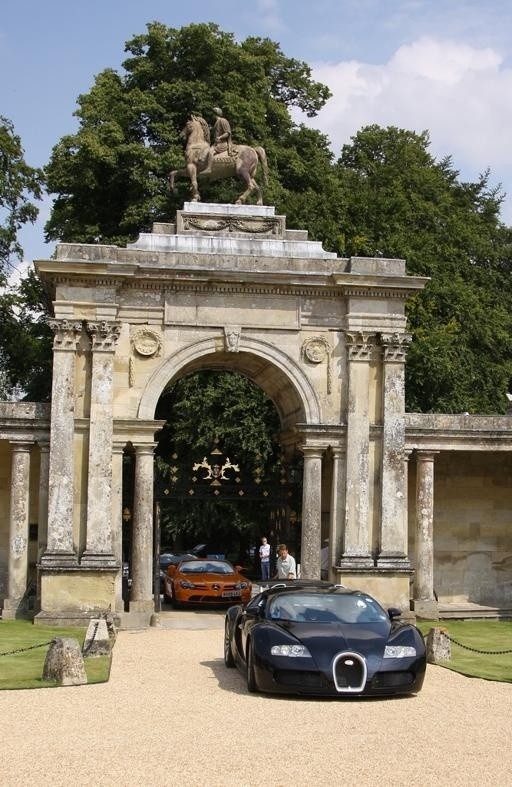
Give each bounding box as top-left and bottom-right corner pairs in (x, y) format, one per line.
(320, 536), (331, 581)
(258, 536), (272, 580)
(273, 543), (297, 579)
(198, 106), (237, 175)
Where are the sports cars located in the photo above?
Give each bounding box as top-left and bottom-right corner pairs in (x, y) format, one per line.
(158, 556), (251, 611)
(226, 584), (427, 697)
